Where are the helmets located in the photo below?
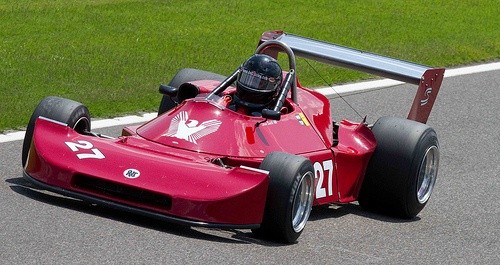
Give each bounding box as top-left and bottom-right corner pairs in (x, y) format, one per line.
(233, 54), (283, 109)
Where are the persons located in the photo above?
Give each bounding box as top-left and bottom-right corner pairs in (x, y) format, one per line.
(227, 53), (283, 117)
